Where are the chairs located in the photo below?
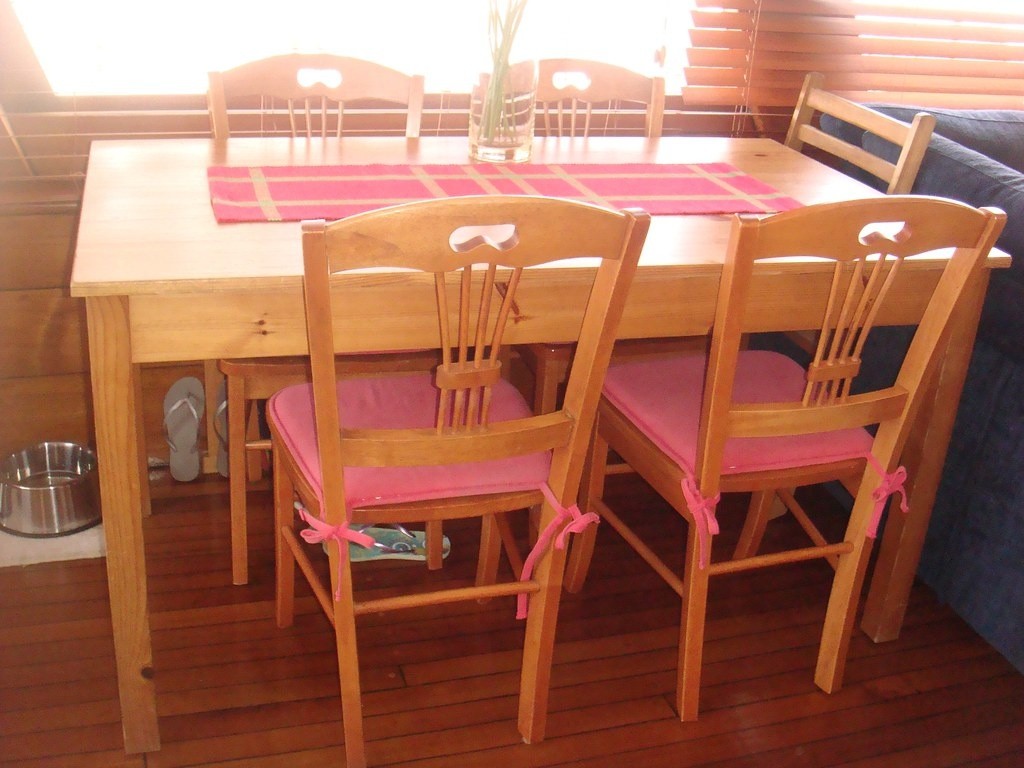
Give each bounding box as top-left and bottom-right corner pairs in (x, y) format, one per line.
(207, 53), (1008, 768)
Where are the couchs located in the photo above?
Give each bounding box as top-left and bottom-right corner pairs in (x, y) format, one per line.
(745, 102), (1023, 666)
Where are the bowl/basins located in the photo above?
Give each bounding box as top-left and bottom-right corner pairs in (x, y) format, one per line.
(0, 442), (102, 539)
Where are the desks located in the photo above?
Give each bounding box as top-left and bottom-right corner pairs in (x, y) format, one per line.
(67, 138), (1011, 754)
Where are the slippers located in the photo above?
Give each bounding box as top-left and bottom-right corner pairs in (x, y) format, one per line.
(321, 521), (451, 563)
(213, 379), (252, 479)
(163, 376), (206, 483)
